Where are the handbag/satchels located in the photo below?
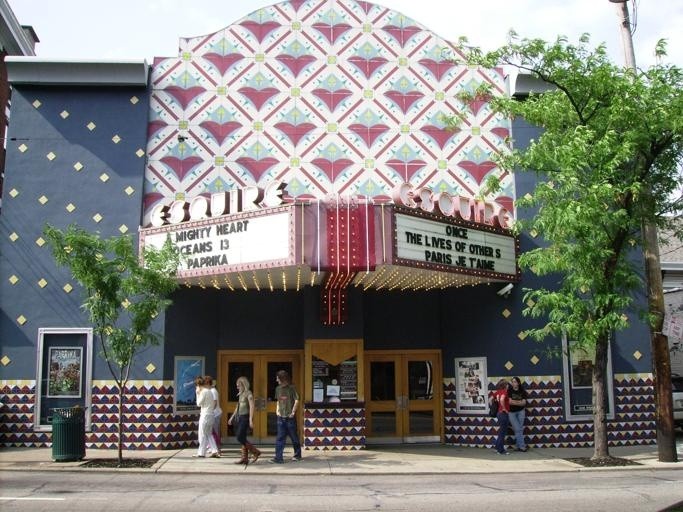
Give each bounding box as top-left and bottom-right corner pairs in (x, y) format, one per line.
(232, 415), (239, 432)
(489, 401), (498, 416)
(213, 408), (222, 417)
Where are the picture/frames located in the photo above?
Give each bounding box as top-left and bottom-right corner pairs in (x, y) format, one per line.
(568, 340), (593, 390)
(46, 346), (84, 400)
(453, 356), (491, 416)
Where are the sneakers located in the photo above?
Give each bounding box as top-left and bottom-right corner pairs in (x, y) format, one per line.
(193, 454), (205, 458)
(210, 453), (220, 458)
(268, 458), (285, 464)
(497, 451), (509, 455)
(292, 457), (304, 461)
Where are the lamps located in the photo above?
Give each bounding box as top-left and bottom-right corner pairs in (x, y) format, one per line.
(497, 280), (515, 298)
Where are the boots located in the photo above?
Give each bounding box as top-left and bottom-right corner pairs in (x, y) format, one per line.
(235, 446), (248, 465)
(246, 444), (261, 463)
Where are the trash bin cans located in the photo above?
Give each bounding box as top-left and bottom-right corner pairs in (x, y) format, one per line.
(49, 406), (88, 462)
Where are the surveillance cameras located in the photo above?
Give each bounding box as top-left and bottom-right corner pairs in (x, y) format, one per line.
(497, 282), (514, 298)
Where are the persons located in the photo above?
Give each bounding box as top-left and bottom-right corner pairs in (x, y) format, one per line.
(192, 376), (220, 458)
(226, 377), (261, 465)
(491, 378), (510, 454)
(266, 369), (301, 463)
(203, 375), (224, 455)
(503, 376), (527, 452)
